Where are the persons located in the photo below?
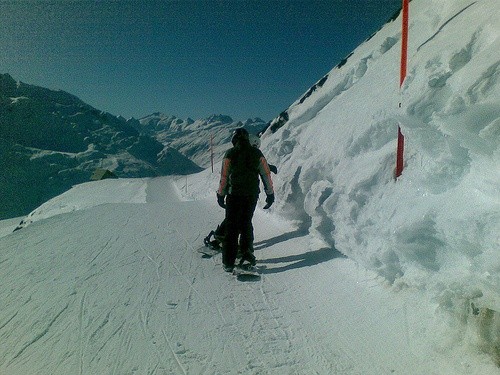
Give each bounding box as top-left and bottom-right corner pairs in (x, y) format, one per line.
(205, 127), (278, 272)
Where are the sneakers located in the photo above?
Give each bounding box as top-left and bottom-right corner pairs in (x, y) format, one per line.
(243, 248), (255, 262)
(222, 264), (233, 272)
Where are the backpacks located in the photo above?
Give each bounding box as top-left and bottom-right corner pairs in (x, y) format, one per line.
(228, 147), (258, 202)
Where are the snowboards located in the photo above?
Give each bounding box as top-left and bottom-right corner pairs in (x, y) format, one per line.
(232, 244), (260, 278)
(197, 240), (222, 257)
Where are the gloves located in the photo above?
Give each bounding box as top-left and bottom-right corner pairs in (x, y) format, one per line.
(217, 193), (226, 208)
(263, 193), (274, 209)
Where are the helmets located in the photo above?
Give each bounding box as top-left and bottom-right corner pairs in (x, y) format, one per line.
(232, 128), (249, 143)
